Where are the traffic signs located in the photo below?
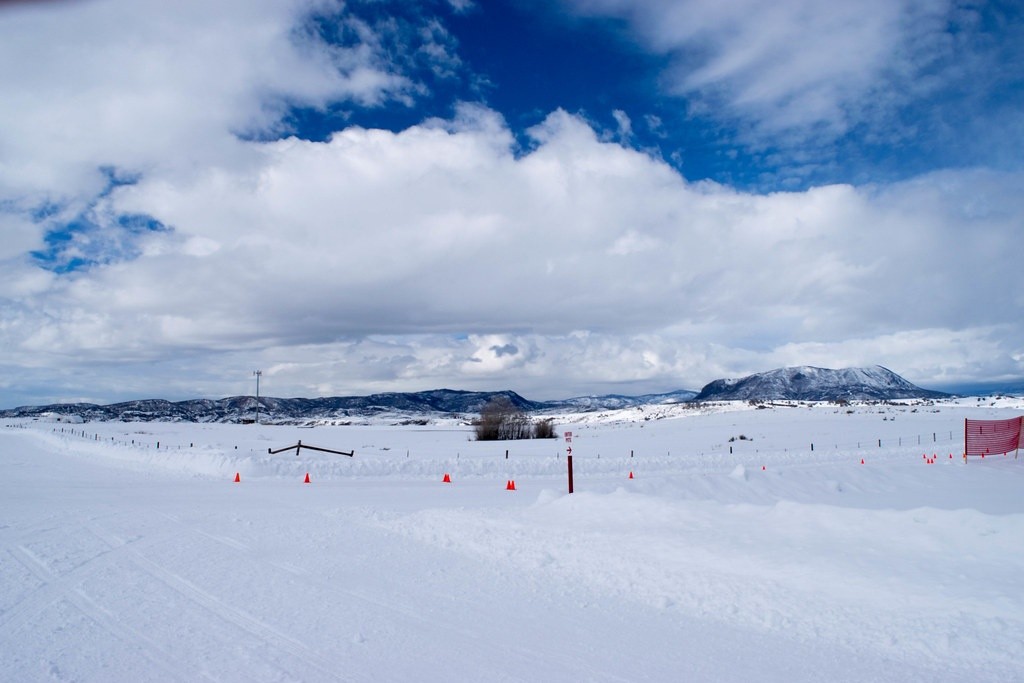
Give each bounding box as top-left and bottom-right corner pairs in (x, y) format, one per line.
(564, 432), (572, 455)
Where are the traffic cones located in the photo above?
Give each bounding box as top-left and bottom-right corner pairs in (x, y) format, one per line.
(443, 474), (451, 482)
(304, 473), (310, 483)
(861, 459), (864, 464)
(762, 466), (765, 470)
(234, 473), (241, 482)
(922, 453), (936, 463)
(629, 472), (633, 478)
(981, 448), (989, 458)
(949, 453), (952, 458)
(506, 481), (515, 490)
